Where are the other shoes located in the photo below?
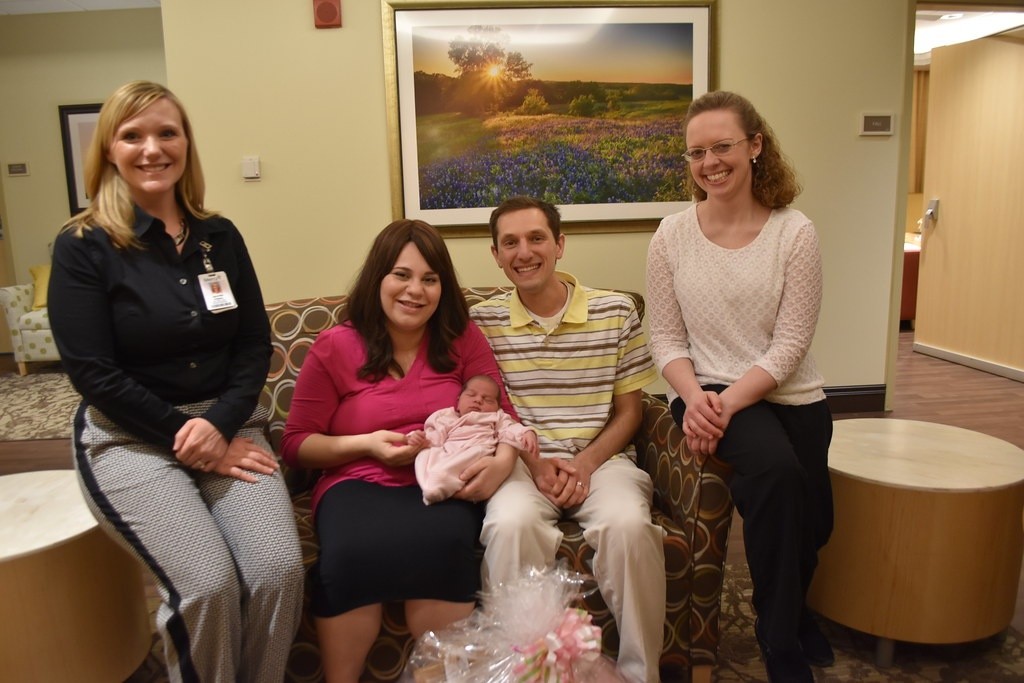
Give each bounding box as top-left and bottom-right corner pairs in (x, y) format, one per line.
(791, 607), (834, 666)
(754, 614), (815, 683)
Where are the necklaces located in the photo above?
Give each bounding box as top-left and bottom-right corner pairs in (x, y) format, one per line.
(173, 217), (187, 246)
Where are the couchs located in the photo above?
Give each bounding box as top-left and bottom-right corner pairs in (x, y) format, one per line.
(259, 290), (733, 683)
(0, 284), (60, 376)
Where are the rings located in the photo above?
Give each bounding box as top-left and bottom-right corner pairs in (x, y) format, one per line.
(197, 460), (205, 469)
(576, 482), (584, 489)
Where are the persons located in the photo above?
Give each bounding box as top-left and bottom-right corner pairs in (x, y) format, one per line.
(282, 220), (519, 683)
(468, 199), (666, 683)
(407, 374), (539, 506)
(47, 82), (304, 683)
(645, 91), (836, 683)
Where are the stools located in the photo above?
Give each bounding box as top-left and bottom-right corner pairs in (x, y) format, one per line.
(809, 417), (1023, 669)
(0, 470), (150, 683)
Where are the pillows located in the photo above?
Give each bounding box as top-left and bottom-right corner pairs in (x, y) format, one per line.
(29, 264), (52, 310)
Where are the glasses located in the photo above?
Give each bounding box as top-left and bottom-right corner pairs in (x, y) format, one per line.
(681, 136), (751, 162)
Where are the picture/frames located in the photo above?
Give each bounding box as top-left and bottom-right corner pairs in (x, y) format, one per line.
(381, 0), (718, 235)
(58, 103), (105, 217)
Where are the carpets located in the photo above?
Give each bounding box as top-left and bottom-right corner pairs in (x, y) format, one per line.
(0, 371), (81, 441)
